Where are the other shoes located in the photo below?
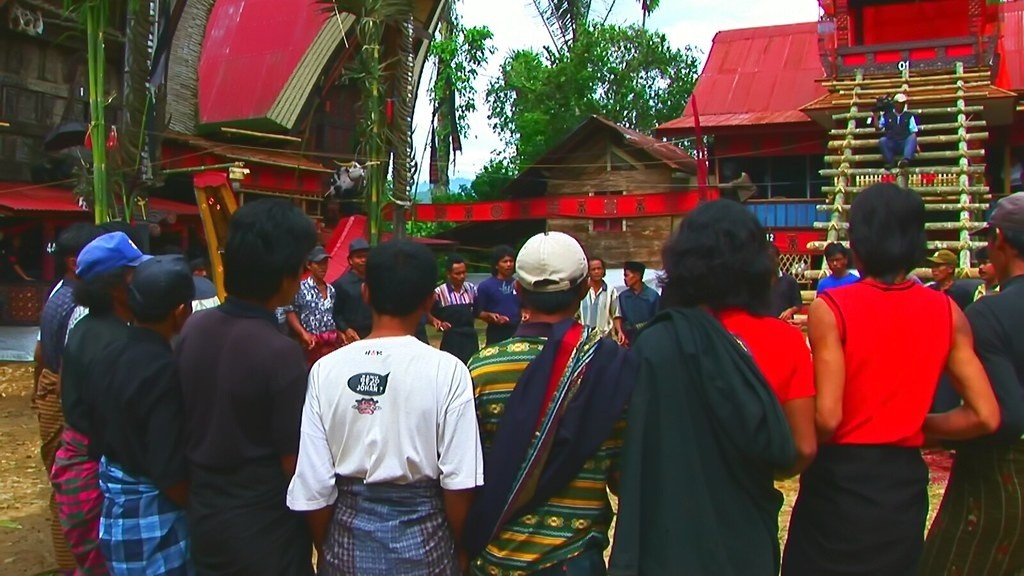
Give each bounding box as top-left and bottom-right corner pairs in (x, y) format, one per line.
(898, 160), (908, 168)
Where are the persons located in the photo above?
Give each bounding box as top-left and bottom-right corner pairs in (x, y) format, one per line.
(925, 250), (973, 311)
(781, 183), (1001, 576)
(974, 245), (1000, 302)
(32, 196), (818, 576)
(0, 231), (35, 280)
(918, 192), (1024, 576)
(872, 92), (918, 169)
(815, 241), (860, 293)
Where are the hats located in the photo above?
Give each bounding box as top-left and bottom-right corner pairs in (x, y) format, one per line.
(515, 229), (588, 292)
(306, 245), (332, 262)
(126, 254), (217, 322)
(894, 94), (907, 102)
(968, 191), (1024, 241)
(925, 250), (958, 266)
(349, 238), (370, 253)
(625, 261), (646, 274)
(75, 231), (154, 289)
